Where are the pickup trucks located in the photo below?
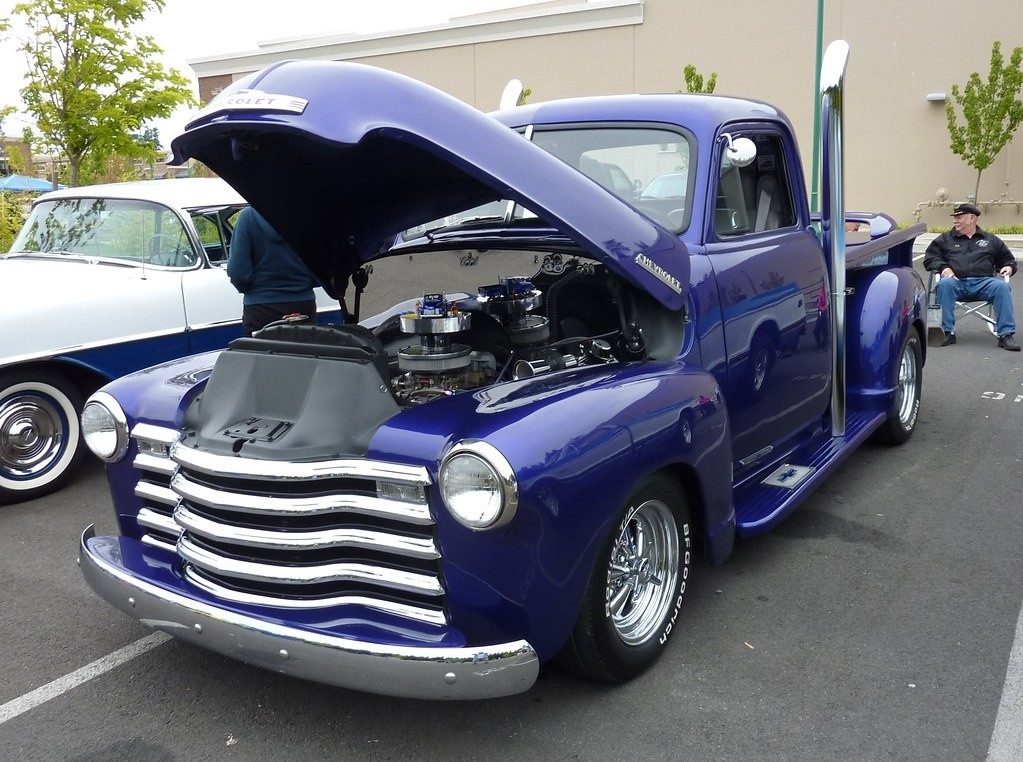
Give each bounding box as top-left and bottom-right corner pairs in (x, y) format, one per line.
(77, 58), (928, 701)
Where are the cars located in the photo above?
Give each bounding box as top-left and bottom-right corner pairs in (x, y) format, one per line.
(0, 176), (405, 502)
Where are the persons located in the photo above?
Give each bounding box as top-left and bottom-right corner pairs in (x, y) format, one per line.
(225, 206), (317, 335)
(923, 204), (1022, 352)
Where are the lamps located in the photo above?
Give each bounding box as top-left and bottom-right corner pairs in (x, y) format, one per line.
(926, 93), (947, 102)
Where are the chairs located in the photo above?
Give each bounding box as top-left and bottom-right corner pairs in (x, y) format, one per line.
(926, 268), (1010, 336)
(716, 158), (783, 239)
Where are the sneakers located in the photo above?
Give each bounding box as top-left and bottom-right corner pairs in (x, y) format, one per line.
(998, 335), (1021, 351)
(941, 331), (956, 346)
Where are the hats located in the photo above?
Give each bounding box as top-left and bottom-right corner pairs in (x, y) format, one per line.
(950, 204), (981, 217)
